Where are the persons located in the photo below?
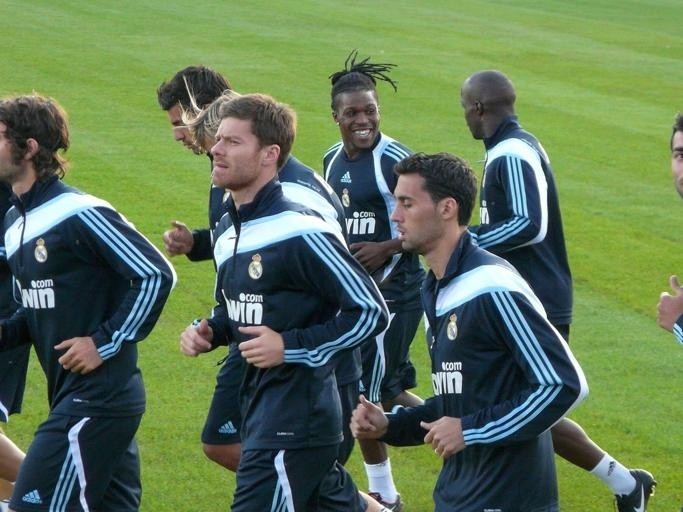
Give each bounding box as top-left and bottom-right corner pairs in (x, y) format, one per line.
(458, 71), (656, 510)
(655, 111), (682, 347)
(348, 151), (591, 512)
(323, 48), (427, 512)
(0, 181), (32, 501)
(156, 67), (364, 474)
(178, 94), (394, 512)
(0, 89), (179, 511)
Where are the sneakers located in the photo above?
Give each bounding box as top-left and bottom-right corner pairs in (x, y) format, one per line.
(615, 469), (656, 512)
(368, 492), (403, 512)
